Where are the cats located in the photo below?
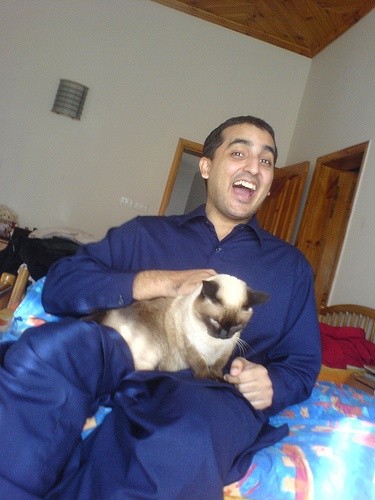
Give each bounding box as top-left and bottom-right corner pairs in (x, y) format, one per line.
(0, 273), (272, 382)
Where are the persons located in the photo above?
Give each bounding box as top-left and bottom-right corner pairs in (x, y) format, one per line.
(0, 116), (322, 500)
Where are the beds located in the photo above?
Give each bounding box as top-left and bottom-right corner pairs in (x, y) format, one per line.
(0, 264), (375, 500)
